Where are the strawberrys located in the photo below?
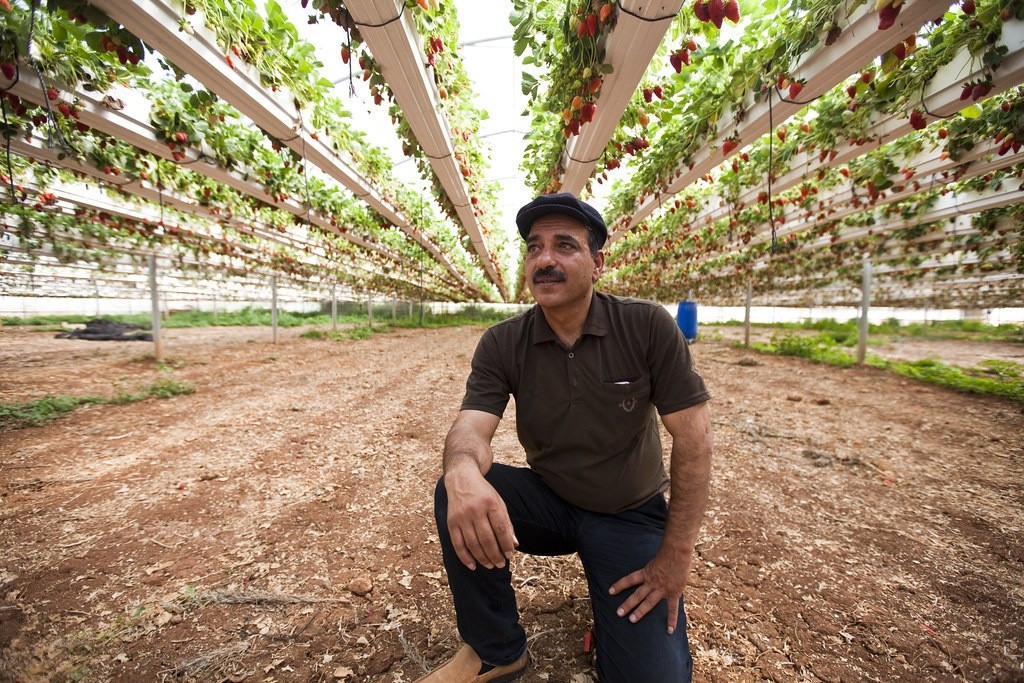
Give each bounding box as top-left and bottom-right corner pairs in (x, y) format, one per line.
(0, 1), (1022, 310)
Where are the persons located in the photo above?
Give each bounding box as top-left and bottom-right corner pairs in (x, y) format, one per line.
(410, 193), (714, 682)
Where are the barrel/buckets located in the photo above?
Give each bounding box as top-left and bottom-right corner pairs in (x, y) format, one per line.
(678, 301), (698, 340)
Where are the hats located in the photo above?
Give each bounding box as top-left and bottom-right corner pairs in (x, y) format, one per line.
(516, 192), (607, 250)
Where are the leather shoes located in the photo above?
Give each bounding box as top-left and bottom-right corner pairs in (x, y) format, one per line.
(413, 643), (530, 682)
